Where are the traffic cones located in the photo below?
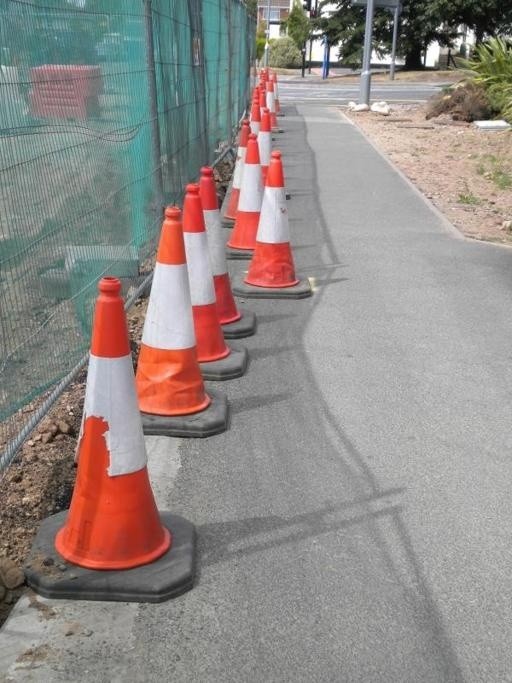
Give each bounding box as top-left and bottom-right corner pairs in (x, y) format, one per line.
(22, 273), (197, 601)
(225, 132), (267, 261)
(182, 165), (257, 337)
(127, 207), (229, 438)
(217, 63), (313, 226)
(172, 185), (247, 377)
(234, 149), (315, 299)
